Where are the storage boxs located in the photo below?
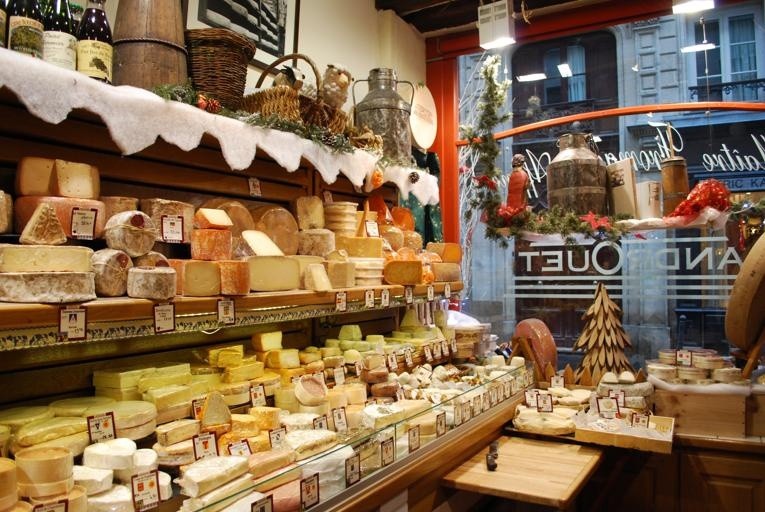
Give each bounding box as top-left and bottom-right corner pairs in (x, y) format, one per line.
(653, 387), (765, 440)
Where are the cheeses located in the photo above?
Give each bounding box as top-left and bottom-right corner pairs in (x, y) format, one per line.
(513, 346), (751, 440)
(0, 154), (526, 512)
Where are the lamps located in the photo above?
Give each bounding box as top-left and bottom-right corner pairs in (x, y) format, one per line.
(477, 0), (517, 51)
(672, 0), (715, 16)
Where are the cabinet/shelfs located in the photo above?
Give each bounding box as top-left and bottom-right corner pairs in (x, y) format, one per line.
(573, 433), (765, 512)
(0, 45), (537, 512)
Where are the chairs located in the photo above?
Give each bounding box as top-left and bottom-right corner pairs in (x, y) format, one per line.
(512, 318), (558, 381)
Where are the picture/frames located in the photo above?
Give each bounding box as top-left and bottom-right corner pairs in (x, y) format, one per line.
(179, 0), (301, 77)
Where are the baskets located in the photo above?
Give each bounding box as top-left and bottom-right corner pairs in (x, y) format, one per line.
(241, 54), (348, 134)
(184, 28), (255, 112)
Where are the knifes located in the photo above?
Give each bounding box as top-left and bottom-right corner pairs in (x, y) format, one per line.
(485, 438), (502, 470)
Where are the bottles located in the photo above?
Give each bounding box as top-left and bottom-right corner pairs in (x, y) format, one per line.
(0, 0), (6, 48)
(5, 0), (43, 60)
(75, 0), (113, 85)
(42, 0), (75, 72)
